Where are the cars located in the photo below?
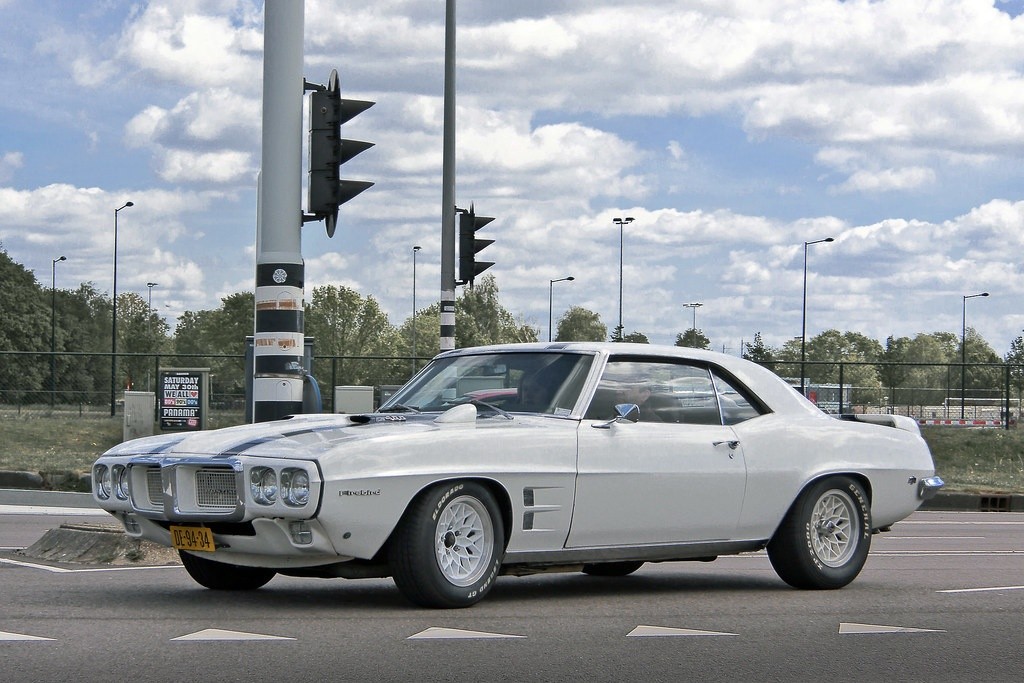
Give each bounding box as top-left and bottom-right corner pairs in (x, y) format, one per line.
(84, 341), (943, 608)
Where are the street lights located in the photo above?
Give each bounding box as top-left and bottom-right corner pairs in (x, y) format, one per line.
(611, 216), (635, 339)
(801, 237), (835, 396)
(146, 282), (158, 392)
(959, 292), (989, 418)
(411, 245), (422, 376)
(548, 276), (575, 342)
(108, 202), (135, 416)
(50, 256), (67, 406)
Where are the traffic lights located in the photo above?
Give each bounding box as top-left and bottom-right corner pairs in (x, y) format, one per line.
(306, 68), (376, 240)
(458, 201), (495, 292)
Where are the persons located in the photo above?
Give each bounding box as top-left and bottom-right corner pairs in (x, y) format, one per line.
(605, 369), (668, 422)
